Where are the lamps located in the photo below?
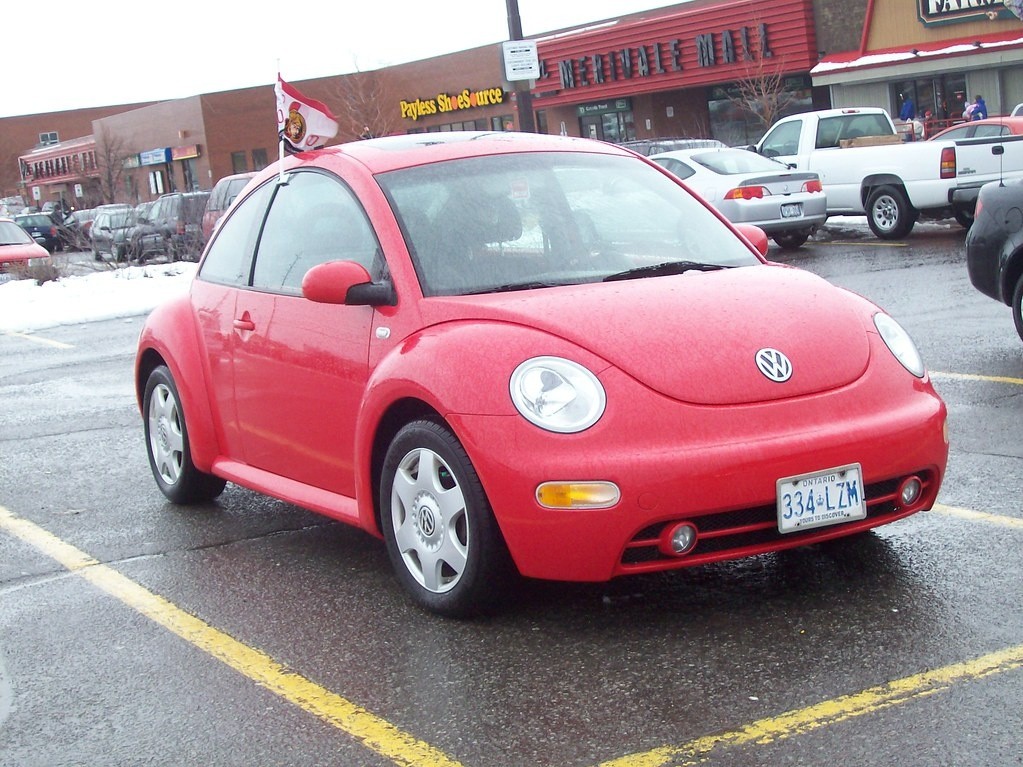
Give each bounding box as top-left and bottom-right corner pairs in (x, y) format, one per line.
(972, 40), (981, 47)
(910, 49), (919, 56)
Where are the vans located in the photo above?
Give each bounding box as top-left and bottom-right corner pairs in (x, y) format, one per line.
(203, 170), (261, 242)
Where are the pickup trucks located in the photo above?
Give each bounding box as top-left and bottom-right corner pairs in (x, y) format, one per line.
(746, 108), (1023, 240)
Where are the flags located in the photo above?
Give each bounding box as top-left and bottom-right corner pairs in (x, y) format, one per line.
(274, 77), (340, 154)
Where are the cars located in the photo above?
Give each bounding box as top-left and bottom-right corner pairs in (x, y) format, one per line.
(0, 219), (51, 275)
(135, 131), (949, 619)
(922, 116), (1023, 136)
(64, 203), (159, 265)
(643, 148), (828, 248)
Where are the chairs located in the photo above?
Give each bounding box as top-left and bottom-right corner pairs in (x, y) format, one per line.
(279, 203), (371, 288)
(434, 186), (541, 286)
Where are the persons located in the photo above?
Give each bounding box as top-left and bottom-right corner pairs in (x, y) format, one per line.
(962, 94), (987, 122)
(897, 91), (934, 142)
(54, 198), (71, 252)
(938, 100), (955, 127)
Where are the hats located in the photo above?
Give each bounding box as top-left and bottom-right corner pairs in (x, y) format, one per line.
(925, 111), (932, 116)
(965, 101), (970, 108)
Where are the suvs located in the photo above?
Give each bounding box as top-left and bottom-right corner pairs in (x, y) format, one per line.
(131, 192), (212, 264)
(15, 214), (58, 254)
